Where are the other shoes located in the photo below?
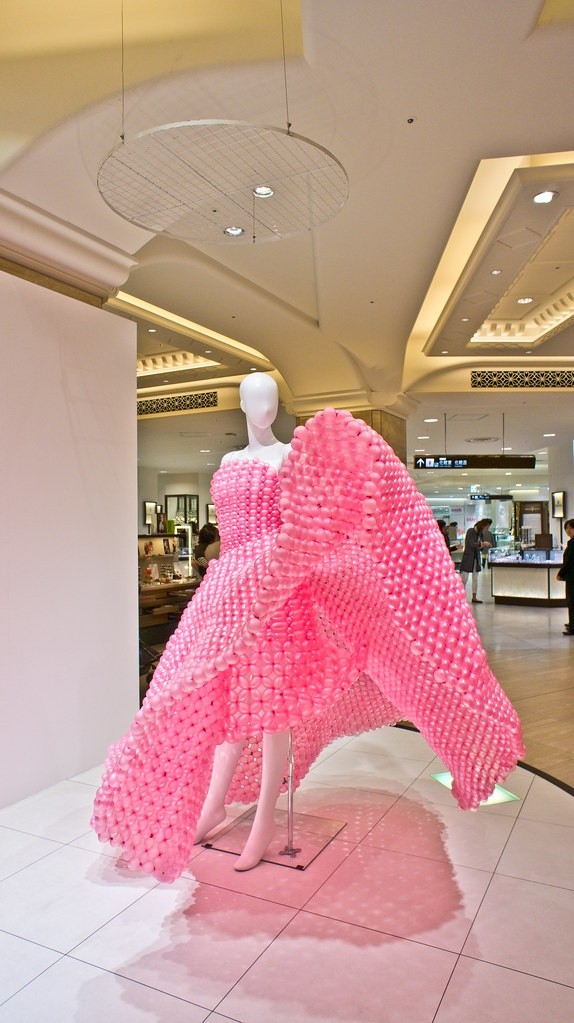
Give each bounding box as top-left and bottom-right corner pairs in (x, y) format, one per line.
(472, 599), (482, 603)
(563, 631), (574, 635)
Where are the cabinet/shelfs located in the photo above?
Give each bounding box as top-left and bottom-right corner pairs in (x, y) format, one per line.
(138, 533), (200, 628)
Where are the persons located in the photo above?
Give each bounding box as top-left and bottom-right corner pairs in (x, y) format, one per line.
(557, 518), (574, 635)
(91, 369), (527, 885)
(459, 521), (493, 604)
(447, 522), (458, 539)
(480, 518), (497, 570)
(188, 521), (221, 576)
(437, 519), (463, 556)
